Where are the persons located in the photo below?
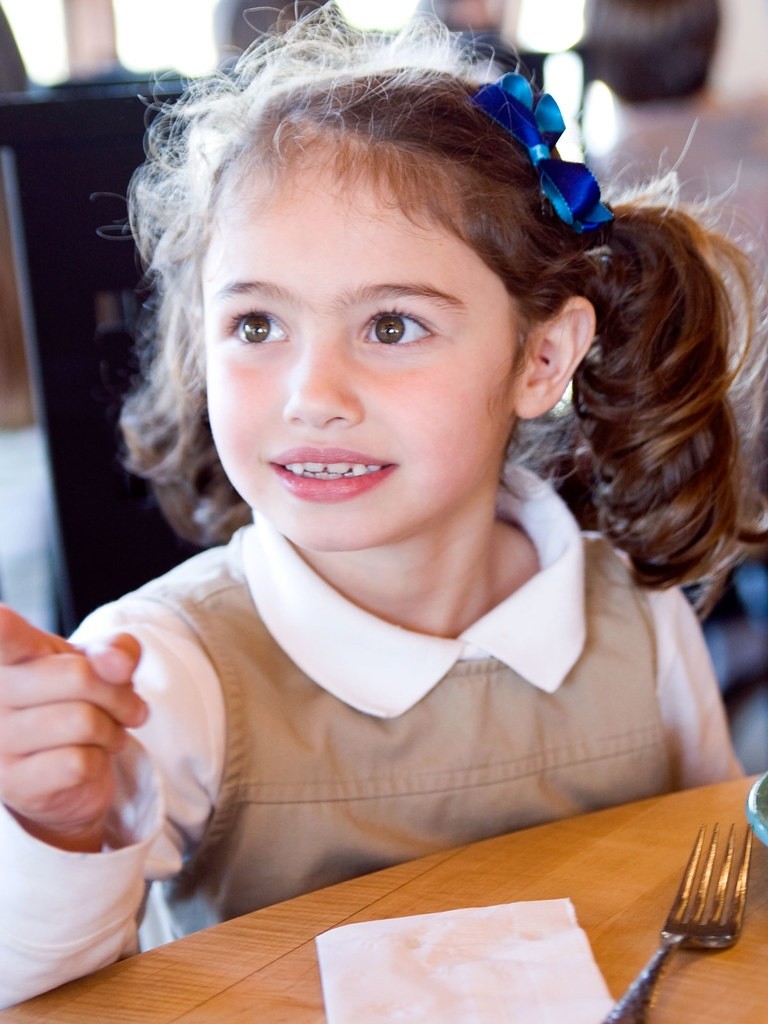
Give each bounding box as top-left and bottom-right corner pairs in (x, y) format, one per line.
(0, 6), (768, 1013)
(542, 1), (768, 630)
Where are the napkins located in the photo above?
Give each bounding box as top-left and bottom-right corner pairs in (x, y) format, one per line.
(313, 900), (619, 1024)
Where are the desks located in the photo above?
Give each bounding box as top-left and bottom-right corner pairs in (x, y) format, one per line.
(0, 774), (768, 1024)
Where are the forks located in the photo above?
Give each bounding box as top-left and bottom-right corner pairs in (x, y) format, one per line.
(603, 823), (753, 1024)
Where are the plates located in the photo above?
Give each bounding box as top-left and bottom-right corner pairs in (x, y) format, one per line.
(745, 770), (768, 849)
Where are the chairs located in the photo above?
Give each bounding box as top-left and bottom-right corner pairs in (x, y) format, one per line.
(0, 70), (249, 648)
